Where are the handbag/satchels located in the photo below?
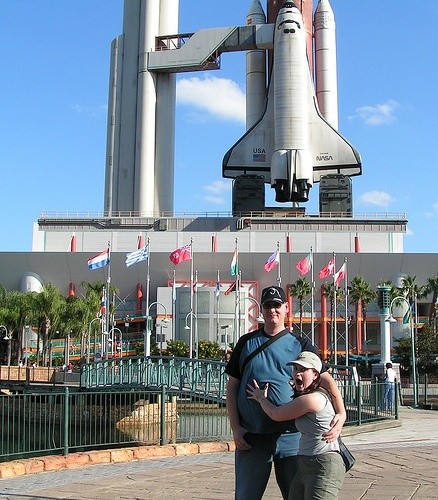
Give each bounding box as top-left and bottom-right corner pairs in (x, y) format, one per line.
(339, 442), (356, 472)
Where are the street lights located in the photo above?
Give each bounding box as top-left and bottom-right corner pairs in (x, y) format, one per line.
(184, 312), (197, 360)
(384, 296), (418, 408)
(146, 302), (167, 356)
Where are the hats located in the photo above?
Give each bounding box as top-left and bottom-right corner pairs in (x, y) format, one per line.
(261, 286), (286, 305)
(286, 351), (322, 374)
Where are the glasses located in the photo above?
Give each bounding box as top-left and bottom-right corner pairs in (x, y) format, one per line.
(262, 303), (283, 309)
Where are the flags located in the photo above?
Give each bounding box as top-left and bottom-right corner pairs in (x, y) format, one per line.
(335, 262), (345, 287)
(100, 296), (107, 315)
(403, 304), (414, 324)
(264, 250), (278, 273)
(230, 251), (237, 276)
(296, 253), (312, 275)
(318, 257), (334, 280)
(169, 245), (190, 265)
(87, 248), (110, 270)
(125, 245), (148, 268)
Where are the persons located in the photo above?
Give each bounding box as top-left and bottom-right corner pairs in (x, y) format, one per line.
(19, 361), (23, 367)
(246, 351), (346, 500)
(67, 363), (73, 370)
(32, 362), (36, 367)
(224, 286), (347, 500)
(381, 363), (397, 413)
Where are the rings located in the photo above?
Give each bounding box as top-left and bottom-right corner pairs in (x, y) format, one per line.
(239, 443), (240, 444)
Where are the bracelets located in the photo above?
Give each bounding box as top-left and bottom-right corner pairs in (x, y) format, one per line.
(259, 398), (266, 403)
